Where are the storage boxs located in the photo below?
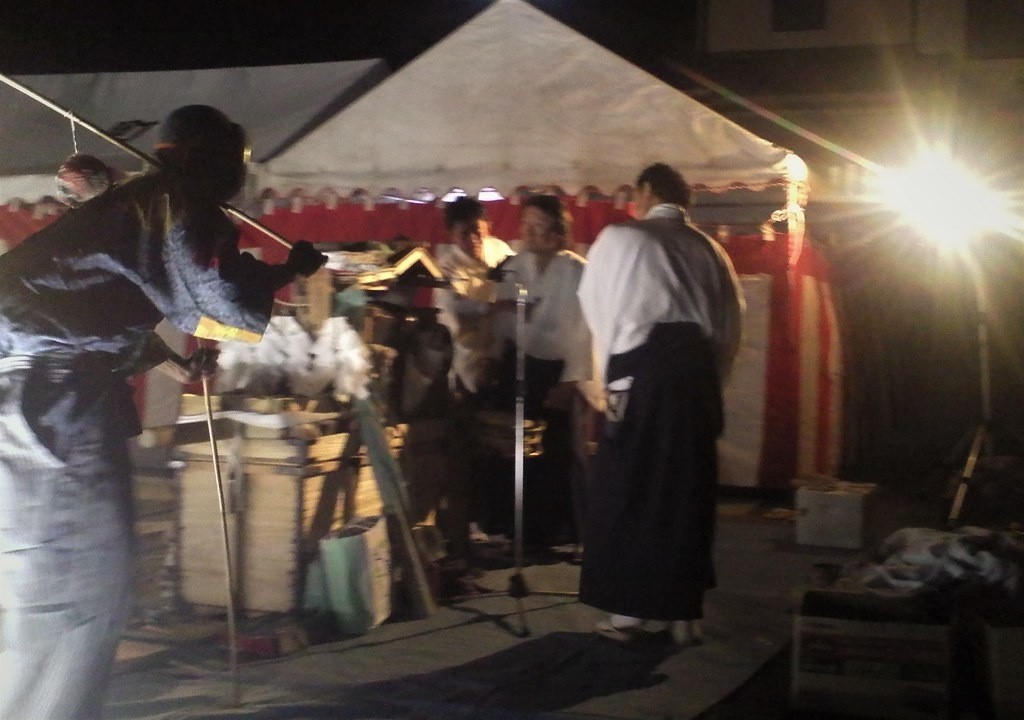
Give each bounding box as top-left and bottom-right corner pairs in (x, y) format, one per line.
(173, 423), (438, 612)
(797, 481), (877, 549)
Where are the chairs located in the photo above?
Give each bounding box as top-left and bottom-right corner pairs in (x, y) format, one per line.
(792, 424), (985, 720)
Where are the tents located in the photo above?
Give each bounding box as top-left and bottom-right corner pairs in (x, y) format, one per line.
(245, 3), (842, 490)
(1, 59), (410, 219)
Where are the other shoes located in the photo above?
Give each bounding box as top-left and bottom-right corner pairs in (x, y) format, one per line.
(593, 618), (636, 642)
(546, 545), (583, 564)
(505, 540), (532, 564)
(430, 548), (467, 570)
(651, 628), (705, 647)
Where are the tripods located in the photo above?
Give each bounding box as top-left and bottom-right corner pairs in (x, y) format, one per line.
(435, 285), (580, 637)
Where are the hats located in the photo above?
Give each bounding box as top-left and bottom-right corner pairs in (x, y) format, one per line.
(150, 104), (246, 152)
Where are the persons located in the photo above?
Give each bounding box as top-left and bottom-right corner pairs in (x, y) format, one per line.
(55, 153), (592, 558)
(0, 105), (329, 720)
(575, 161), (742, 648)
